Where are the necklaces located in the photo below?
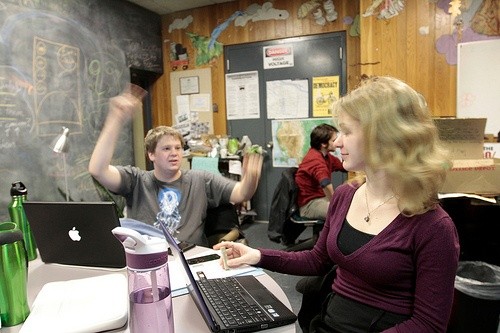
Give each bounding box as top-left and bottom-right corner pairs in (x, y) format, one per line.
(360, 180), (400, 226)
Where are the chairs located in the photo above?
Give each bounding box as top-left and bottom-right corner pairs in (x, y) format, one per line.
(205, 202), (247, 246)
(293, 256), (500, 333)
(269, 169), (324, 252)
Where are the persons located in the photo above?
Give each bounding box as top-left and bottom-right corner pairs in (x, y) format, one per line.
(295, 121), (352, 220)
(213, 75), (459, 333)
(86, 91), (264, 246)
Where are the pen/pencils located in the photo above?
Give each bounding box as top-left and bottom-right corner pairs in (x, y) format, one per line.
(220, 240), (230, 270)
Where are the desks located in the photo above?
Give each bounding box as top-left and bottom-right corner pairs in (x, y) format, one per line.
(434, 196), (500, 263)
(0, 236), (296, 333)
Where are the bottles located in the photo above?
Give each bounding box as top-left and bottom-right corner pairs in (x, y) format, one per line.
(112, 227), (174, 333)
(229, 137), (238, 153)
(241, 136), (251, 156)
(8, 181), (37, 261)
(0, 222), (30, 327)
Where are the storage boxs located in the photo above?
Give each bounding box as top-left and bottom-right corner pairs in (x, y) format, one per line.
(434, 117), (500, 197)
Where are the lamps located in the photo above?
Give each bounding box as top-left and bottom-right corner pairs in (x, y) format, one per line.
(48, 126), (72, 202)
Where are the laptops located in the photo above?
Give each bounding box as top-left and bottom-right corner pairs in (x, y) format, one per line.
(155, 216), (298, 333)
(22, 201), (127, 268)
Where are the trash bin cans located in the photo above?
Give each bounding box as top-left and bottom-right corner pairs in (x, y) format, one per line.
(448, 261), (500, 333)
